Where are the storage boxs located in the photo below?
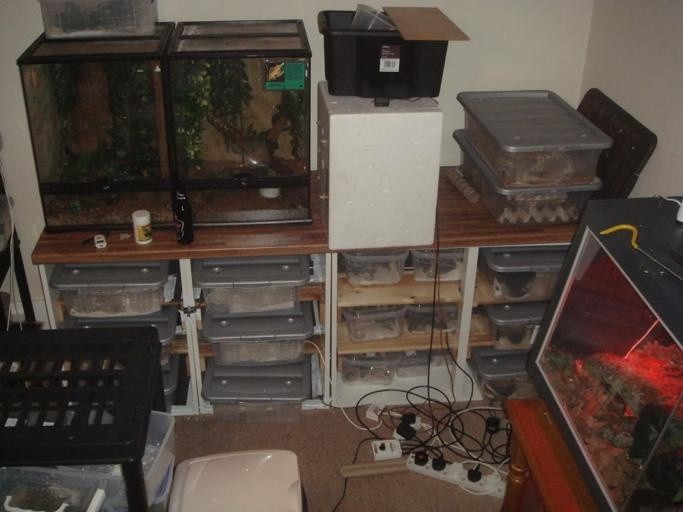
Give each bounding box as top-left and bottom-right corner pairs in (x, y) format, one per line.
(453, 83), (611, 228)
(317, 9), (446, 99)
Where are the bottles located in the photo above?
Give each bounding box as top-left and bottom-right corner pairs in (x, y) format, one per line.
(132, 211), (152, 245)
(174, 184), (192, 245)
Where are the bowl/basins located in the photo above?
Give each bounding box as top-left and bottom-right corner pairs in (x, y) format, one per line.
(348, 4), (395, 32)
(258, 188), (280, 199)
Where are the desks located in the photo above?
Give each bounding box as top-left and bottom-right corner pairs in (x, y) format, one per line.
(498, 397), (601, 510)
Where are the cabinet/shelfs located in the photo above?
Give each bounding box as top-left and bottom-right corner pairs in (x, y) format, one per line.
(30, 242), (601, 413)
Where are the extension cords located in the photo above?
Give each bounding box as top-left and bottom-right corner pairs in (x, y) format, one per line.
(406, 453), (507, 499)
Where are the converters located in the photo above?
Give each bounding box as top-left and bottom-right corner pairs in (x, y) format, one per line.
(398, 423), (415, 438)
(486, 416), (498, 432)
